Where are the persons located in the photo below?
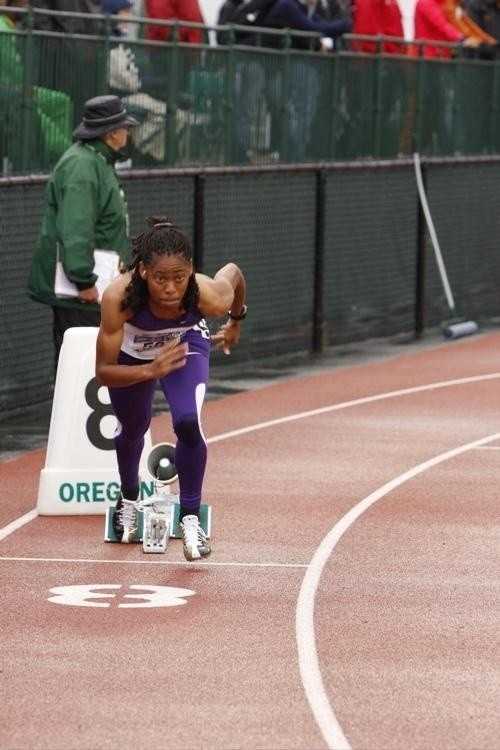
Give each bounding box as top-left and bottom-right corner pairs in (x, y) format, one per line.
(95, 214), (247, 562)
(0, 0), (500, 178)
(27, 93), (140, 387)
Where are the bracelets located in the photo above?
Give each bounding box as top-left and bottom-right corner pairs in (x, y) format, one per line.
(227, 305), (247, 321)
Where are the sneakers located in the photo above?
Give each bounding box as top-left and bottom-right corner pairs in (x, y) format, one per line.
(180, 514), (210, 561)
(113, 492), (140, 544)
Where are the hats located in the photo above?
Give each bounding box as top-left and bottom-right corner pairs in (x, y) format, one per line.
(72, 95), (139, 138)
